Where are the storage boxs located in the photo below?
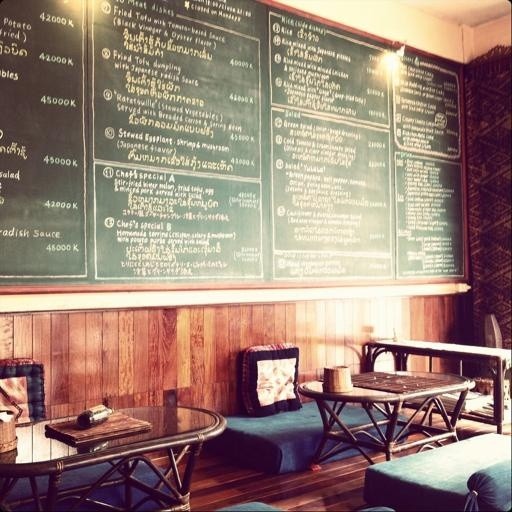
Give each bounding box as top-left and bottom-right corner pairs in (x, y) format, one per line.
(366, 434), (511, 511)
(209, 402), (408, 472)
(215, 500), (288, 511)
(1, 458), (182, 512)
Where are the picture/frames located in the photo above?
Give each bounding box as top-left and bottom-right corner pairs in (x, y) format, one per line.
(298, 370), (474, 472)
(0, 404), (229, 511)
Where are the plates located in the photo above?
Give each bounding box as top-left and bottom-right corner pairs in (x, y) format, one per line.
(366, 339), (512, 433)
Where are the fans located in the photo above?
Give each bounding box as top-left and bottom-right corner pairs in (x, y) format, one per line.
(0, 0), (469, 295)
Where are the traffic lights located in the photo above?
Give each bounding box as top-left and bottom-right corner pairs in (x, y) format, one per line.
(466, 463), (511, 511)
(0, 359), (46, 426)
(241, 343), (305, 416)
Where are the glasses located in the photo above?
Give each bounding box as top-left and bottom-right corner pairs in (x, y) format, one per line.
(392, 39), (407, 58)
